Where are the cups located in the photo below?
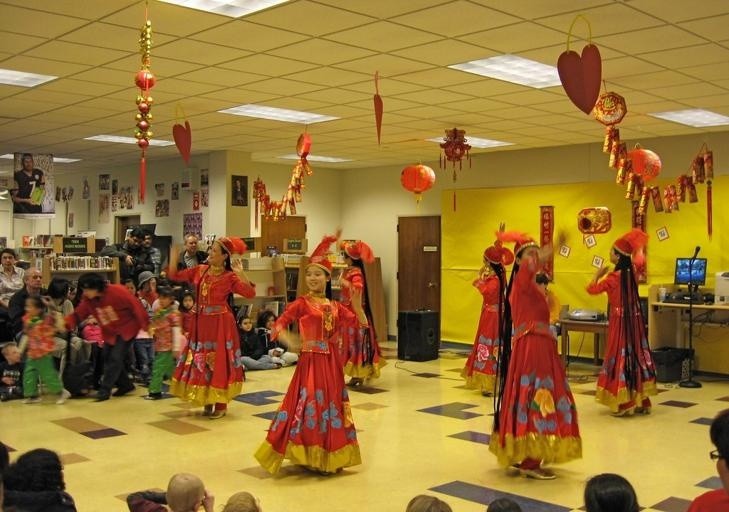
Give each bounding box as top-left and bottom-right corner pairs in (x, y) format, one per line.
(657, 287), (666, 302)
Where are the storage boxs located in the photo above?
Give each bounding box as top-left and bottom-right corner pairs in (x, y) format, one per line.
(651, 345), (695, 383)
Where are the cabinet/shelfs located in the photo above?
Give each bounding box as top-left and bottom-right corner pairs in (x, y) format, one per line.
(229, 250), (386, 358)
(12, 232), (120, 302)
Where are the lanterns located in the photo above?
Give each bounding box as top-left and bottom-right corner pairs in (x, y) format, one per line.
(616, 141), (663, 188)
(400, 159), (438, 205)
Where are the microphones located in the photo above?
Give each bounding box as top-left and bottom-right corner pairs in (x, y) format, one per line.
(692, 245), (701, 263)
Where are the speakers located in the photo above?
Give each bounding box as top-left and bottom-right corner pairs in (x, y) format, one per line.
(395, 306), (439, 362)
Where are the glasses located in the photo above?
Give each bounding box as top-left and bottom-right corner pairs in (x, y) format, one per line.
(3, 255), (12, 260)
(32, 275), (41, 279)
(133, 239), (142, 243)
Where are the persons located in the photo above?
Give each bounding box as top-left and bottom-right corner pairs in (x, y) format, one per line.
(486, 234), (585, 480)
(584, 472), (640, 512)
(232, 178), (246, 205)
(0, 440), (265, 512)
(586, 239), (658, 417)
(15, 153), (46, 213)
(1, 217), (300, 405)
(166, 235), (257, 420)
(253, 256), (372, 477)
(684, 408), (729, 512)
(459, 246), (517, 397)
(484, 497), (523, 511)
(534, 274), (563, 343)
(333, 241), (384, 387)
(405, 494), (454, 512)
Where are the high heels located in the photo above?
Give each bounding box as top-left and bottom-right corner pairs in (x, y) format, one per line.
(346, 377), (363, 387)
(522, 469), (556, 481)
(203, 404), (212, 415)
(610, 405), (636, 417)
(637, 407), (651, 414)
(210, 410), (227, 420)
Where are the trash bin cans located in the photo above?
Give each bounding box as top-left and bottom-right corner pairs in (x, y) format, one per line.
(651, 346), (685, 383)
(677, 348), (695, 380)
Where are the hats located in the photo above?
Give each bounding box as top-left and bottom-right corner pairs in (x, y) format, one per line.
(485, 247), (502, 264)
(614, 238), (632, 256)
(515, 239), (539, 254)
(131, 228), (144, 238)
(344, 242), (360, 260)
(217, 237), (233, 255)
(305, 259), (332, 273)
(138, 271), (157, 288)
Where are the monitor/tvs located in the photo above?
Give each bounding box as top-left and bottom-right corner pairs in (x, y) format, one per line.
(673, 258), (707, 292)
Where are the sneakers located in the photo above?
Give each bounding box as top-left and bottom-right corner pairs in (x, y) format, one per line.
(21, 398), (42, 403)
(145, 393), (161, 400)
(56, 388), (72, 404)
(87, 389), (111, 401)
(113, 385), (135, 396)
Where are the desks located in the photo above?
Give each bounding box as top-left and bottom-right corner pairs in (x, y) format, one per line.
(646, 283), (728, 368)
(559, 317), (612, 375)
